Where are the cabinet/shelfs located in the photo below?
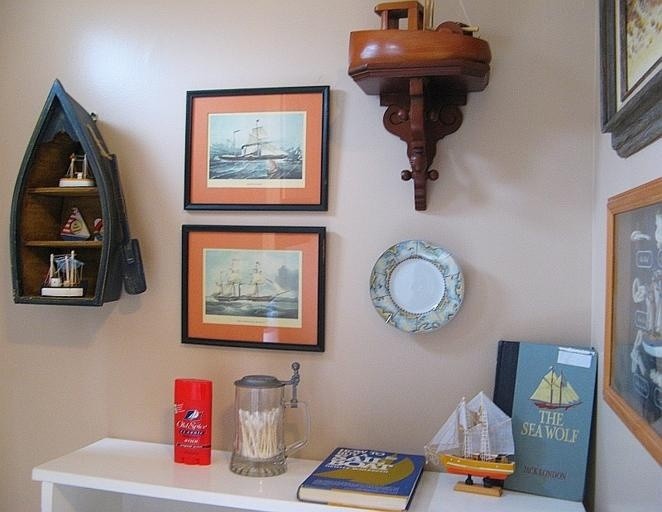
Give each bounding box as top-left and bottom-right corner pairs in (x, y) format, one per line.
(8, 80), (127, 306)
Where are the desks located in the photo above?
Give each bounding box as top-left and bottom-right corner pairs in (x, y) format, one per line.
(33, 438), (586, 512)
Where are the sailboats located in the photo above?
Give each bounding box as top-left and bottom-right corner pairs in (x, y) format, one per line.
(528, 366), (585, 410)
(218, 119), (288, 161)
(210, 258), (293, 303)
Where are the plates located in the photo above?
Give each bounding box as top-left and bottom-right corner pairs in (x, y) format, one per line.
(369, 240), (466, 337)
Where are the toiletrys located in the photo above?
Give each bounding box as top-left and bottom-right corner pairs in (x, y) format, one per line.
(174, 378), (212, 465)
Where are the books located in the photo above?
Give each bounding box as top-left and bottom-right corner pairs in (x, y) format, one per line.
(491, 340), (598, 501)
(297, 446), (427, 511)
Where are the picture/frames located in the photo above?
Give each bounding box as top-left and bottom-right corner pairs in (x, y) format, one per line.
(184, 86), (329, 212)
(601, 176), (662, 470)
(181, 224), (326, 353)
(598, 0), (662, 158)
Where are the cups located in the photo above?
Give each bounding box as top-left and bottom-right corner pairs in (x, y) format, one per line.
(229, 362), (310, 477)
